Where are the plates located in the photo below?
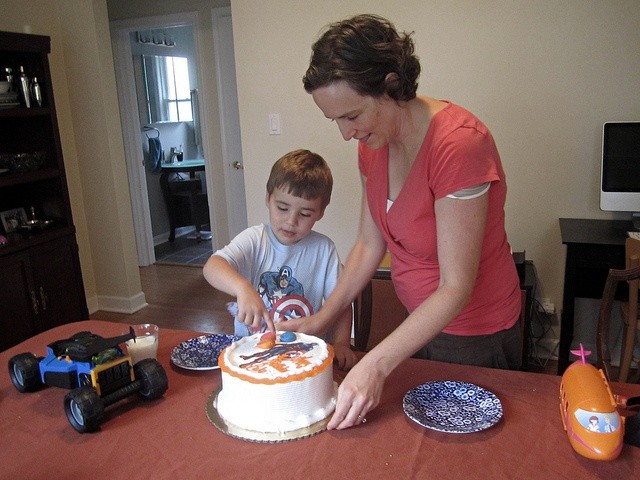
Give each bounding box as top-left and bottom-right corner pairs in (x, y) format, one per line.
(403, 380), (504, 435)
(170, 334), (244, 370)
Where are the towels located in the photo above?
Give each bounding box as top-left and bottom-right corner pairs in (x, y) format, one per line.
(147, 137), (165, 172)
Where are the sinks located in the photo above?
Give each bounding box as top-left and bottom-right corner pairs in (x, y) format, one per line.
(161, 160), (205, 167)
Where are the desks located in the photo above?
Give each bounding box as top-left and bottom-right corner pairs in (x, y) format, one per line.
(160, 161), (205, 241)
(557, 218), (639, 375)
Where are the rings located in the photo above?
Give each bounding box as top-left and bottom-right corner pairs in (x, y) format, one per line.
(358, 415), (368, 424)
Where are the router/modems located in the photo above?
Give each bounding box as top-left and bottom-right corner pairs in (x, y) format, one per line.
(537, 302), (558, 315)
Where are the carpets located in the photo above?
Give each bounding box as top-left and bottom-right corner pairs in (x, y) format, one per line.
(155, 225), (213, 267)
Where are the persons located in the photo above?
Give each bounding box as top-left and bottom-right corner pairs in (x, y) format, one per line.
(202, 149), (358, 374)
(274, 13), (525, 433)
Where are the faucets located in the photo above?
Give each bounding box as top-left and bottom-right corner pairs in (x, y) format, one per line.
(170, 147), (181, 163)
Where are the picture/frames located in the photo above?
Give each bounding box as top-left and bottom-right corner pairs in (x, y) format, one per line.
(0, 207), (28, 233)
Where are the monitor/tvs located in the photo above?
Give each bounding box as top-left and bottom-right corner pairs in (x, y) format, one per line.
(599, 121), (640, 216)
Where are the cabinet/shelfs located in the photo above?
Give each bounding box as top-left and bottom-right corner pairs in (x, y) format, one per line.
(1, 53), (89, 354)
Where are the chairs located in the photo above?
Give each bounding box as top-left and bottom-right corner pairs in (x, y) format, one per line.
(354, 275), (534, 366)
(617, 231), (640, 383)
(597, 265), (640, 383)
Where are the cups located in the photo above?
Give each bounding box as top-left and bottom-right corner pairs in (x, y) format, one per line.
(176, 149), (184, 162)
(123, 325), (159, 365)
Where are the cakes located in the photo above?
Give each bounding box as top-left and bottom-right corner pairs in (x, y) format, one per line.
(215, 329), (339, 433)
